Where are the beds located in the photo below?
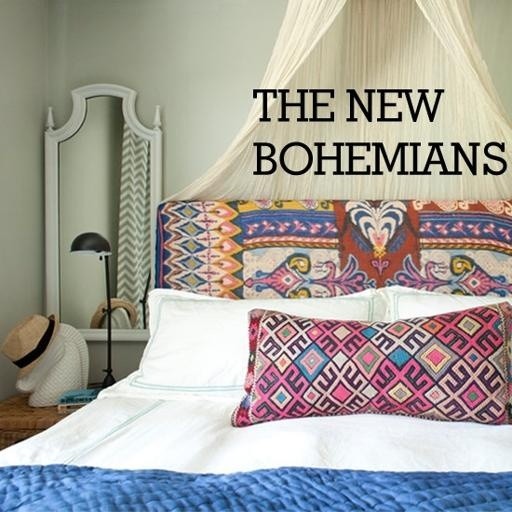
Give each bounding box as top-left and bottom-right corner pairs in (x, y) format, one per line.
(0, 201), (512, 512)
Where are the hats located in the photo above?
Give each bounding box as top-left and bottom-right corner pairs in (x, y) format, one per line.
(0, 314), (59, 379)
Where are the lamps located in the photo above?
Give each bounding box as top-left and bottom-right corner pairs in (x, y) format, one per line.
(68, 233), (115, 388)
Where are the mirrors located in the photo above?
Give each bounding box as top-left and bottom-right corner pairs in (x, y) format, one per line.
(44, 84), (163, 342)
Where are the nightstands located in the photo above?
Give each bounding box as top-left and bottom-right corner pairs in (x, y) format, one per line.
(0, 393), (73, 449)
